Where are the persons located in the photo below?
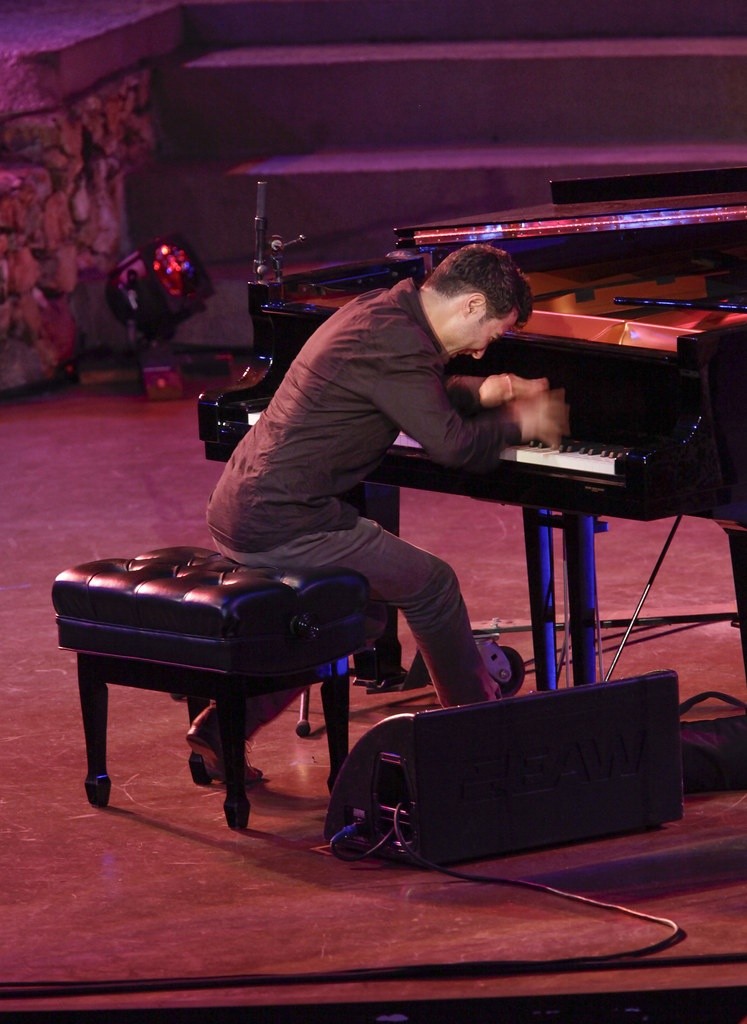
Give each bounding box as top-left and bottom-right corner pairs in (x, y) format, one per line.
(188, 244), (569, 788)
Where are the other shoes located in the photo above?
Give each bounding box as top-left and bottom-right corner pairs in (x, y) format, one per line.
(185, 725), (265, 782)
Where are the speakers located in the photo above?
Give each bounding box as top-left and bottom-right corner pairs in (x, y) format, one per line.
(325, 670), (686, 871)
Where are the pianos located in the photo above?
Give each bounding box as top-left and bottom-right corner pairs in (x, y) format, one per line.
(195, 162), (746, 691)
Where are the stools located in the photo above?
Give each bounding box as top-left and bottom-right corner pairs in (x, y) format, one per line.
(52, 544), (380, 832)
